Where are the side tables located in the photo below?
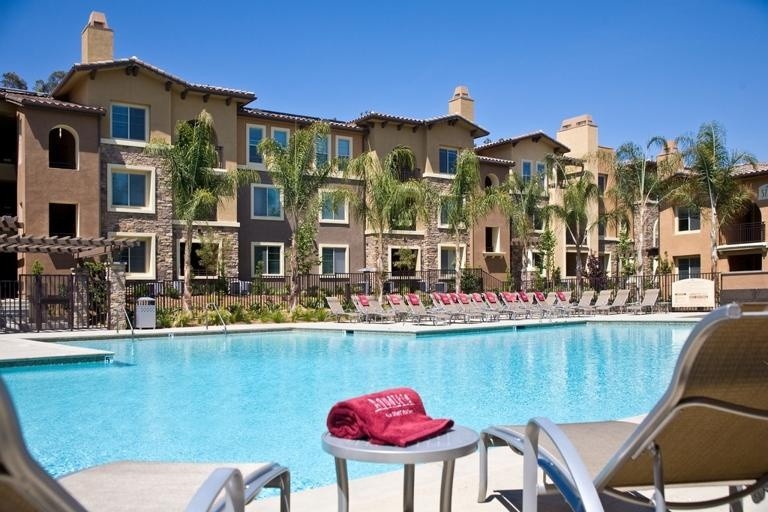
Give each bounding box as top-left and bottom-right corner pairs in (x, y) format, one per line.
(321, 418), (479, 511)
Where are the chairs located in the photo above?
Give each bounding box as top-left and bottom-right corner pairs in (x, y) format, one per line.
(477, 302), (768, 512)
(0, 376), (291, 512)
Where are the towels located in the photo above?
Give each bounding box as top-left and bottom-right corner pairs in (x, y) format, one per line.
(326, 387), (455, 448)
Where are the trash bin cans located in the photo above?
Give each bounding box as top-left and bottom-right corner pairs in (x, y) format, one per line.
(134, 297), (157, 329)
